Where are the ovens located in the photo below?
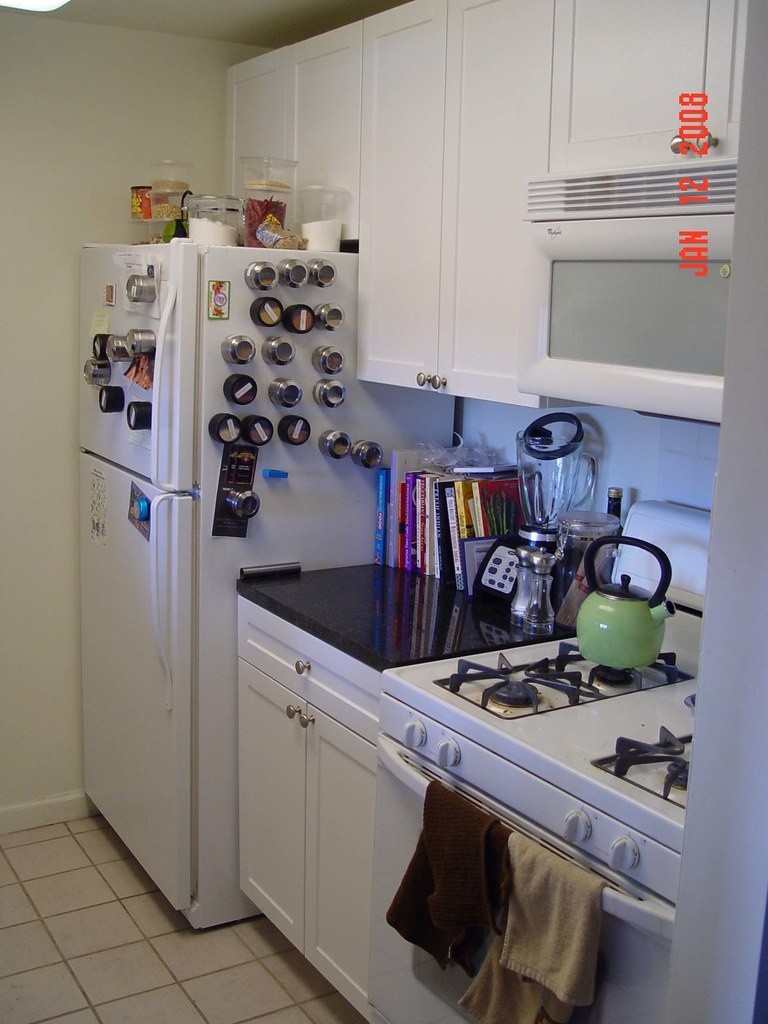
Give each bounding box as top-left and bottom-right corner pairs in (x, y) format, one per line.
(365, 735), (676, 1023)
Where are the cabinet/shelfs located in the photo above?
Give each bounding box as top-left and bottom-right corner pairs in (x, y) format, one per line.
(229, 0), (745, 408)
(236, 596), (382, 1023)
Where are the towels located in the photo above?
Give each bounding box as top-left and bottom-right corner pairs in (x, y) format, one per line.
(455, 832), (610, 1024)
(383, 780), (513, 972)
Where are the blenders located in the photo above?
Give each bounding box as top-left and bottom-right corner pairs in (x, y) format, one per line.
(468, 411), (597, 630)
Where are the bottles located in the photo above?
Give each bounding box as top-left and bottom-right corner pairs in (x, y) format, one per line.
(556, 510), (620, 614)
(510, 546), (539, 627)
(131, 186), (151, 219)
(607, 486), (623, 533)
(523, 548), (556, 636)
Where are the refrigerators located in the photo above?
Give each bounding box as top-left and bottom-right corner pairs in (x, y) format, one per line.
(77, 240), (455, 932)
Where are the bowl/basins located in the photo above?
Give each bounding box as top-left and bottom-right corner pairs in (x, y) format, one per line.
(145, 159), (192, 244)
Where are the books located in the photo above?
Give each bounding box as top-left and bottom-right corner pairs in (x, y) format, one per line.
(373, 447), (544, 594)
(373, 562), (511, 662)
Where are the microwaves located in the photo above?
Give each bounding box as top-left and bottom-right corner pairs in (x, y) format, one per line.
(517, 159), (737, 423)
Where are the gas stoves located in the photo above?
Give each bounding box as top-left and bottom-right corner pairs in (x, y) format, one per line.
(382, 500), (710, 855)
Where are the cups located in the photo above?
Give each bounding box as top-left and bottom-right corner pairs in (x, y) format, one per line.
(186, 193), (240, 248)
(240, 155), (297, 248)
(299, 185), (348, 252)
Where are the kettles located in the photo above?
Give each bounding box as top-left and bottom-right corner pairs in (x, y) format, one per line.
(576, 535), (677, 669)
